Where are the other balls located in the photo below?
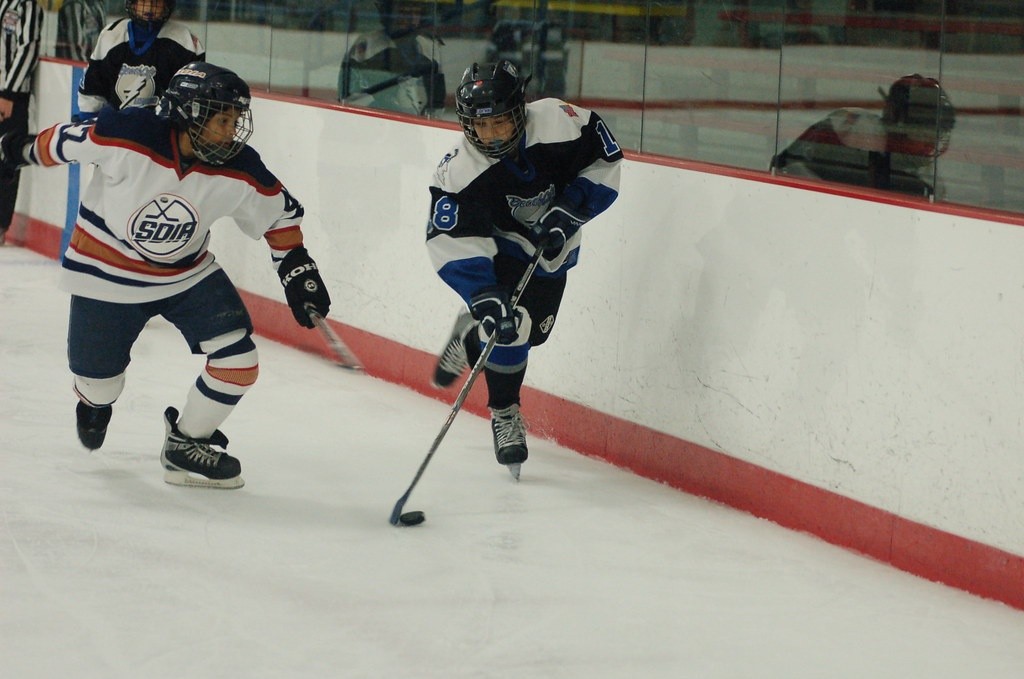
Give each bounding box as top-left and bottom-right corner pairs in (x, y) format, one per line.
(401, 510), (425, 526)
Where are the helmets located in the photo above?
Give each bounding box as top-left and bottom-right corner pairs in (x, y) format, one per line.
(452, 60), (529, 118)
(874, 74), (955, 138)
(154, 59), (251, 130)
(126, 0), (176, 23)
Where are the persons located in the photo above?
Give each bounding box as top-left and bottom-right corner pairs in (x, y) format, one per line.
(340, 0), (955, 201)
(0, 59), (331, 488)
(56, 0), (107, 60)
(0, 0), (204, 247)
(423, 59), (625, 478)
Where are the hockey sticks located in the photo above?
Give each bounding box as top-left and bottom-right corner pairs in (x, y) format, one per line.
(390, 232), (548, 526)
(304, 302), (366, 373)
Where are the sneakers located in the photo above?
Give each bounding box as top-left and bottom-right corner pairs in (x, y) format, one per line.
(487, 404), (529, 482)
(75, 398), (112, 452)
(158, 405), (244, 490)
(426, 306), (475, 388)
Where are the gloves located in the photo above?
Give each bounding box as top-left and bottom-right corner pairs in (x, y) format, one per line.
(534, 201), (586, 249)
(468, 290), (520, 345)
(0, 127), (35, 172)
(281, 251), (332, 329)
